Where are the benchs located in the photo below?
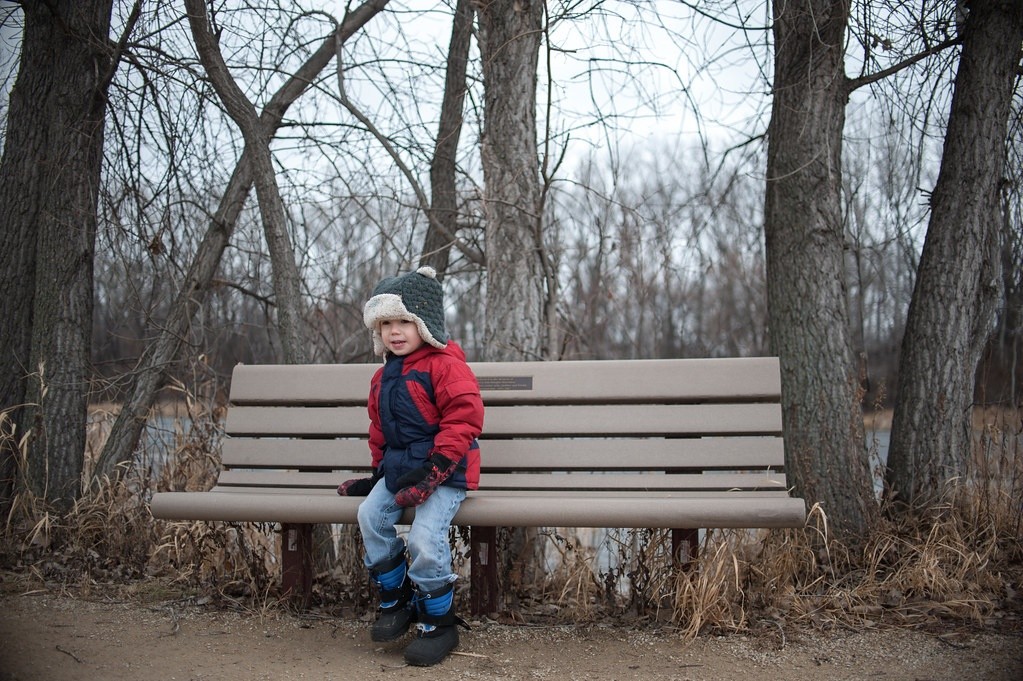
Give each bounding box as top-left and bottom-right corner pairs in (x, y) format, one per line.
(149, 355), (806, 607)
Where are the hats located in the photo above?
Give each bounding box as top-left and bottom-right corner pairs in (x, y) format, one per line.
(363, 266), (447, 356)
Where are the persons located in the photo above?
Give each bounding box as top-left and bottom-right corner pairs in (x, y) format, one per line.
(338, 266), (484, 667)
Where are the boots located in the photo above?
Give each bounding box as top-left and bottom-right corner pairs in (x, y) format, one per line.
(405, 583), (471, 666)
(367, 546), (418, 641)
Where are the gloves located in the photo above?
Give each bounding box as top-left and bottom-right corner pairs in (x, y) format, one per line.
(394, 453), (458, 507)
(337, 476), (380, 496)
